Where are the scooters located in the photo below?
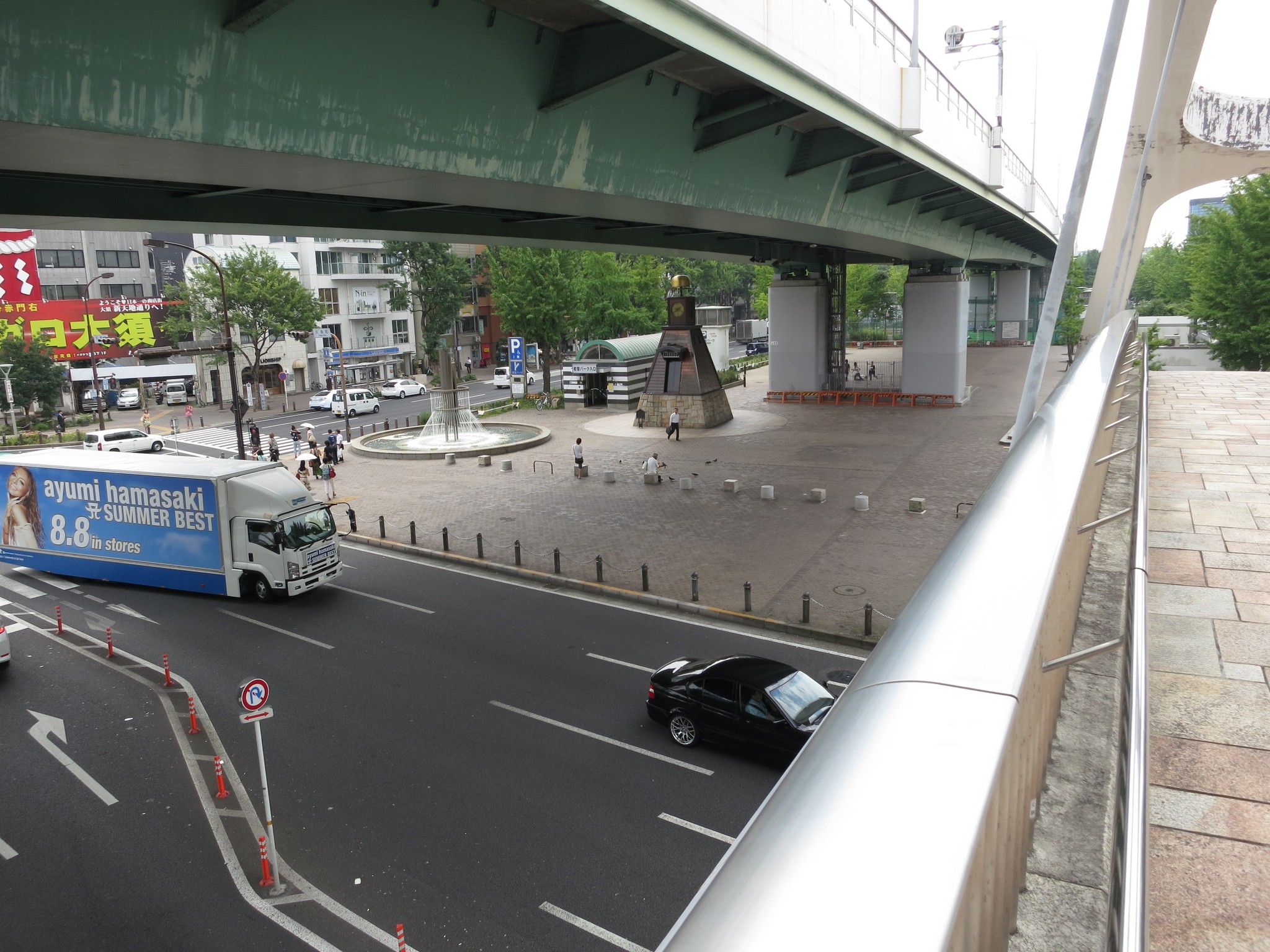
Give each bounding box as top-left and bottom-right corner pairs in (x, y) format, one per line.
(156, 391), (163, 404)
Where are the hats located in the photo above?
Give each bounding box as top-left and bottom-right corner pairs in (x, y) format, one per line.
(185, 403), (189, 405)
(221, 452), (224, 455)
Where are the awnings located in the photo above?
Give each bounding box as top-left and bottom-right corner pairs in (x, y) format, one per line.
(501, 345), (508, 347)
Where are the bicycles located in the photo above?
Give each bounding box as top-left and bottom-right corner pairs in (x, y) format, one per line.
(540, 351), (572, 366)
(338, 373), (358, 384)
(311, 378), (323, 390)
(367, 385), (379, 394)
(535, 392), (552, 411)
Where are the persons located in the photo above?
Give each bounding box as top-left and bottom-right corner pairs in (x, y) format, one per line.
(851, 362), (862, 380)
(668, 408), (681, 441)
(869, 361), (877, 381)
(980, 324), (983, 328)
(290, 425), (344, 501)
(839, 359), (850, 381)
(991, 325), (995, 336)
(184, 402), (194, 429)
(572, 438), (583, 479)
(57, 410), (67, 432)
(647, 453), (663, 480)
(143, 409), (150, 433)
(465, 357), (471, 374)
(253, 450), (267, 461)
(267, 432), (279, 460)
(3, 466), (47, 549)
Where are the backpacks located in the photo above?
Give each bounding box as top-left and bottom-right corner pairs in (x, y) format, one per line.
(270, 438), (278, 450)
(312, 448), (318, 463)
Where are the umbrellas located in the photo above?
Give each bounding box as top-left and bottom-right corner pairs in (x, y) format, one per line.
(300, 422), (315, 433)
(295, 453), (318, 469)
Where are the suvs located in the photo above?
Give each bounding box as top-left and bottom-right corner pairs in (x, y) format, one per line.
(83, 428), (166, 453)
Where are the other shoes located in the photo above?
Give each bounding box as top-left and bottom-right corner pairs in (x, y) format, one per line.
(334, 463), (336, 465)
(578, 477), (580, 479)
(336, 462), (338, 464)
(339, 460), (342, 461)
(676, 439), (680, 441)
(668, 435), (670, 439)
(316, 477), (318, 479)
(295, 456), (297, 458)
(342, 460), (344, 462)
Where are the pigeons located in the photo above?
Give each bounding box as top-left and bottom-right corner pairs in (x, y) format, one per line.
(641, 460), (645, 464)
(619, 459), (622, 464)
(691, 473), (699, 477)
(712, 458), (717, 462)
(704, 461), (712, 465)
(662, 462), (668, 469)
(668, 476), (676, 482)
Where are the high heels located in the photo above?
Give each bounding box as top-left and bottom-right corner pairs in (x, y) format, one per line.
(327, 497), (331, 500)
(333, 493), (336, 498)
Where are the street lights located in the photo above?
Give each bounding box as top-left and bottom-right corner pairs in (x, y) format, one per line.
(84, 273), (116, 429)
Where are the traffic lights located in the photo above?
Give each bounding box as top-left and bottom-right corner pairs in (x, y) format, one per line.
(288, 329), (309, 338)
(99, 337), (120, 344)
(250, 426), (260, 445)
(110, 378), (116, 389)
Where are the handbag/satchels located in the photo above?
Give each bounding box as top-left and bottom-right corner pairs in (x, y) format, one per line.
(337, 448), (342, 458)
(308, 435), (316, 442)
(666, 426), (672, 435)
(328, 464), (335, 477)
(338, 444), (344, 450)
(642, 462), (648, 470)
(326, 453), (333, 462)
(56, 426), (63, 432)
(575, 458), (584, 464)
(143, 420), (151, 428)
(140, 417), (144, 422)
(309, 460), (313, 467)
(298, 435), (301, 440)
(296, 473), (300, 480)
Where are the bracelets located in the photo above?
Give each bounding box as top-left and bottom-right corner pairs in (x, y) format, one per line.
(3, 529), (8, 535)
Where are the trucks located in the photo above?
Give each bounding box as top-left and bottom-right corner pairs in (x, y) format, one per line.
(82, 388), (111, 413)
(735, 320), (769, 344)
(0, 447), (358, 604)
(165, 378), (188, 406)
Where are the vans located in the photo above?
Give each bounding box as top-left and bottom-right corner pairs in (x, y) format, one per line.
(746, 342), (768, 356)
(493, 366), (536, 388)
(332, 389), (381, 418)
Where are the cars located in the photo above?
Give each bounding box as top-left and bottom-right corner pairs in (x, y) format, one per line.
(143, 239), (247, 460)
(116, 387), (142, 411)
(381, 379), (427, 399)
(0, 616), (11, 663)
(645, 653), (839, 753)
(309, 389), (335, 411)
